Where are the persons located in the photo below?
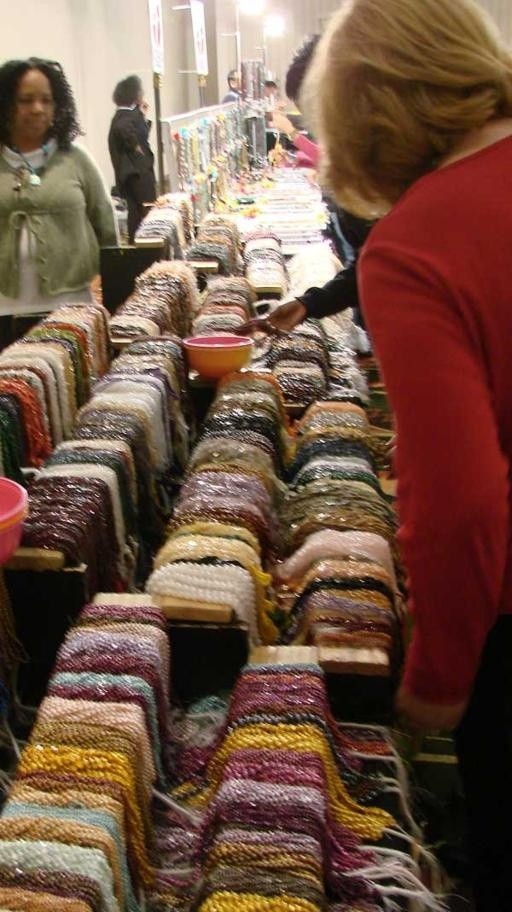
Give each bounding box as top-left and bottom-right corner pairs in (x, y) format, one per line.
(264, 37), (380, 331)
(0, 60), (118, 349)
(297, 0), (512, 912)
(223, 70), (321, 168)
(109, 76), (155, 243)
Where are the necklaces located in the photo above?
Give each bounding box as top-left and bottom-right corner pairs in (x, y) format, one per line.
(10, 138), (49, 186)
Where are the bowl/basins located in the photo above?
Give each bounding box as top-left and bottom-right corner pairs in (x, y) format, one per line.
(182, 334), (256, 380)
(1, 477), (30, 567)
